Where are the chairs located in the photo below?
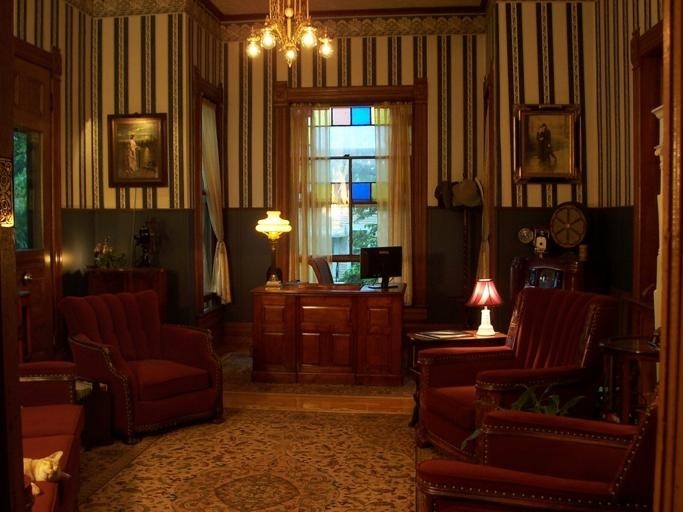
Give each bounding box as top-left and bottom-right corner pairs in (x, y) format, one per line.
(411, 287), (621, 463)
(58, 289), (222, 444)
(414, 380), (659, 512)
(307, 257), (334, 284)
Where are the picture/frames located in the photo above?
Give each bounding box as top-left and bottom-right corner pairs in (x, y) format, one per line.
(512, 104), (582, 185)
(107, 112), (168, 187)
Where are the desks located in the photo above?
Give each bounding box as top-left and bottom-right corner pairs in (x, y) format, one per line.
(84, 266), (178, 324)
(406, 329), (507, 427)
(611, 334), (660, 425)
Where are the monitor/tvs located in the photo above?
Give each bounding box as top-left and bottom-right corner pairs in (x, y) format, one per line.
(361, 246), (402, 289)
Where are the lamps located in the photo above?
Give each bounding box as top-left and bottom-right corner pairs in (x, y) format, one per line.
(93, 231), (113, 262)
(452, 177), (491, 281)
(254, 210), (291, 286)
(463, 279), (505, 335)
(246, 0), (334, 70)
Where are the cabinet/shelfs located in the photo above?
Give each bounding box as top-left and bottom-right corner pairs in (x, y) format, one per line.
(248, 283), (408, 386)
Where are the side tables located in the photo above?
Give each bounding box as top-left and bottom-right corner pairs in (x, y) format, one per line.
(73, 375), (115, 451)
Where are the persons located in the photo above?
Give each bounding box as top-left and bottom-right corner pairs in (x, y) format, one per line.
(537, 123), (557, 164)
(127, 133), (140, 173)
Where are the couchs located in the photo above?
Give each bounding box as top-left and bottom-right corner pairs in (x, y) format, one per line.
(20, 360), (85, 512)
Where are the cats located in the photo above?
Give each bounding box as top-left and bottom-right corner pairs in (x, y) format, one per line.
(23, 450), (72, 496)
